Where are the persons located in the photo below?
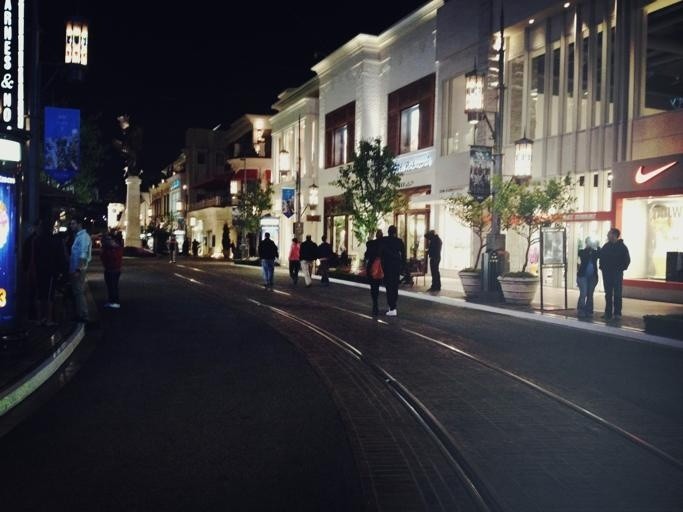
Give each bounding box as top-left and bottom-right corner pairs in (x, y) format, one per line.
(288, 238), (302, 287)
(598, 228), (632, 319)
(257, 232), (278, 287)
(363, 230), (384, 316)
(577, 235), (600, 318)
(424, 230), (442, 292)
(317, 235), (333, 287)
(166, 233), (201, 264)
(300, 236), (318, 288)
(22, 213), (124, 325)
(373, 225), (407, 316)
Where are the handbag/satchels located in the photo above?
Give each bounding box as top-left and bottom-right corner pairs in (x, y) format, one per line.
(371, 257), (385, 280)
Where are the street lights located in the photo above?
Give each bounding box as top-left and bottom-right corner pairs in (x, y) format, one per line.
(24, 3), (88, 326)
(279, 149), (319, 243)
(138, 203), (152, 238)
(464, 49), (534, 301)
(175, 193), (196, 241)
(229, 180), (256, 260)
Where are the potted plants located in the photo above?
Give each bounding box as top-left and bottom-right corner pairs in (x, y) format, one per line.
(490, 171), (581, 307)
(445, 188), (499, 301)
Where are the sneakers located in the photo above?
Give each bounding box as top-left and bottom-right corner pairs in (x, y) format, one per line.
(103, 302), (120, 308)
(385, 307), (397, 316)
